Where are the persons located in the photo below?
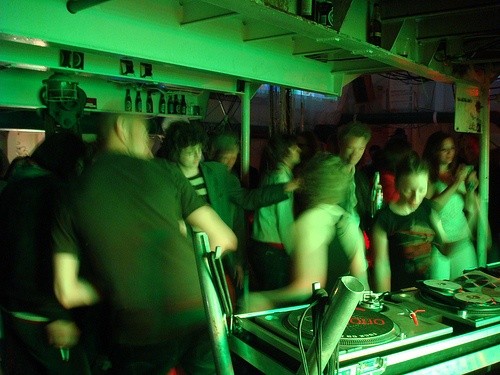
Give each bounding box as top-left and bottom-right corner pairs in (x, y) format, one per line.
(50, 110), (238, 375)
(0, 113), (500, 375)
(0, 129), (90, 375)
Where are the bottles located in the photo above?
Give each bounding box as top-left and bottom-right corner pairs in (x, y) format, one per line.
(173, 94), (180, 114)
(168, 94), (175, 114)
(136, 90), (143, 112)
(375, 185), (384, 215)
(160, 93), (166, 114)
(180, 95), (187, 114)
(147, 88), (153, 113)
(126, 88), (132, 111)
